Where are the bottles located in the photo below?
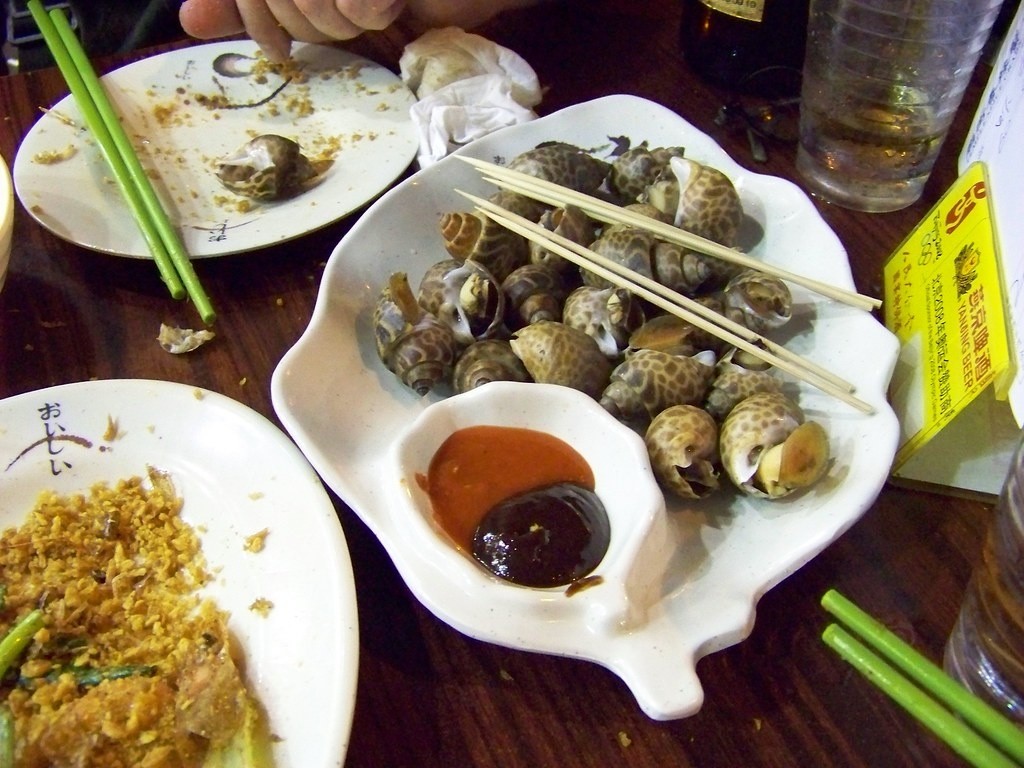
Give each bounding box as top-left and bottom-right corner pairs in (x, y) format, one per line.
(679, 0), (810, 106)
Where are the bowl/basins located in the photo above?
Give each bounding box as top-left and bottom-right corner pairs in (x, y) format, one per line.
(0, 154), (15, 294)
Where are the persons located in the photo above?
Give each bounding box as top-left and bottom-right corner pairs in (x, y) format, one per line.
(119, 0), (538, 59)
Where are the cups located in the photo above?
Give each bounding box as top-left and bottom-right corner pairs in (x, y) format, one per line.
(945, 422), (1024, 731)
(793, 0), (1004, 213)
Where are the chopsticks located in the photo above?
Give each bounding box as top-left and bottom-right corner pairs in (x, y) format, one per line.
(27, 0), (217, 325)
(817, 588), (1024, 768)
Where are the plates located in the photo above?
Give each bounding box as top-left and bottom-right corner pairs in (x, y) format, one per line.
(267, 87), (903, 720)
(12, 39), (421, 262)
(0, 378), (360, 768)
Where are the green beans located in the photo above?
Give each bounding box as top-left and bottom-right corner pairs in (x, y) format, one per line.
(0, 608), (158, 768)
